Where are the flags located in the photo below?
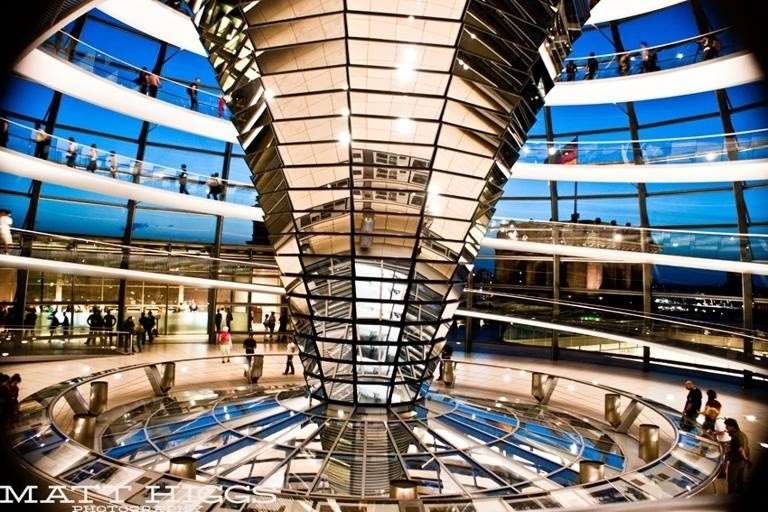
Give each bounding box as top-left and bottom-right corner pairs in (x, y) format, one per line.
(544, 138), (576, 165)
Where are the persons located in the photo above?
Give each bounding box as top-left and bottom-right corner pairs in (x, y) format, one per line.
(0, 300), (297, 430)
(619, 49), (632, 76)
(190, 78), (200, 111)
(585, 51), (601, 80)
(1, 210), (12, 254)
(217, 94), (225, 117)
(682, 381), (702, 432)
(438, 342), (458, 387)
(140, 65), (160, 98)
(697, 389), (721, 435)
(694, 38), (712, 63)
(726, 419), (749, 500)
(0, 117), (226, 199)
(640, 42), (651, 73)
(564, 60), (577, 81)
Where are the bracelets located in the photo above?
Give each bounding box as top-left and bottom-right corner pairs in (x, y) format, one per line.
(683, 411), (688, 416)
(192, 92), (194, 94)
